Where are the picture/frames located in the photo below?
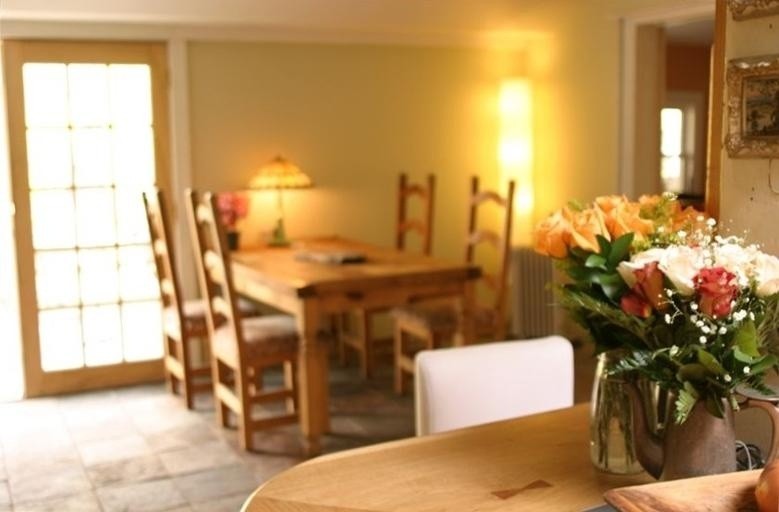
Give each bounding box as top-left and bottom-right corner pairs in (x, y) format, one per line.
(725, 54), (779, 159)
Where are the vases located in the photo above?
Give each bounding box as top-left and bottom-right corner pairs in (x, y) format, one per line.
(660, 397), (779, 481)
(588, 353), (660, 474)
(224, 232), (240, 251)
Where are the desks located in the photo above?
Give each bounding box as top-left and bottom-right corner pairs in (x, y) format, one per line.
(203, 237), (483, 455)
(244, 367), (779, 512)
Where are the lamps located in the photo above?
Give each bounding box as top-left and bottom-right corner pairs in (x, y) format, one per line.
(246, 155), (314, 248)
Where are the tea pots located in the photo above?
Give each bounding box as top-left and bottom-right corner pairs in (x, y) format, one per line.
(619, 379), (778, 483)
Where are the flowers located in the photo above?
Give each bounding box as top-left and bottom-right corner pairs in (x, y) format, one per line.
(544, 215), (779, 425)
(212, 192), (248, 233)
(529, 192), (710, 468)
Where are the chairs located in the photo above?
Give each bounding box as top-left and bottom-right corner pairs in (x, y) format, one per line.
(139, 190), (267, 408)
(387, 170), (518, 398)
(412, 333), (576, 434)
(332, 173), (437, 383)
(184, 191), (331, 452)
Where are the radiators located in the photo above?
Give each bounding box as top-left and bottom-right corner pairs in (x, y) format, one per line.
(503, 242), (557, 339)
